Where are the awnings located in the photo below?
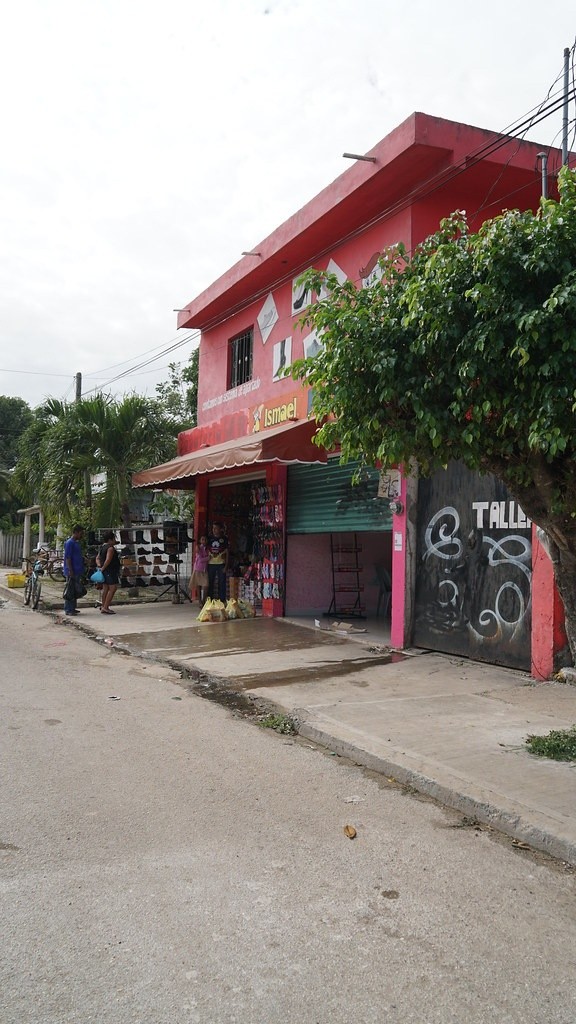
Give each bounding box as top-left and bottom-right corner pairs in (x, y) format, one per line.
(132, 412), (327, 489)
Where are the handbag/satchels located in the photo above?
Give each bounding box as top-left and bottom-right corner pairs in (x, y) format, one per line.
(90, 568), (105, 583)
(196, 597), (256, 622)
(62, 575), (87, 601)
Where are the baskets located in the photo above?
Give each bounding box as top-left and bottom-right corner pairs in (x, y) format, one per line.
(7, 575), (25, 588)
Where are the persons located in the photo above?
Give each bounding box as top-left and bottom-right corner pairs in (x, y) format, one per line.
(189, 522), (230, 609)
(64, 526), (84, 616)
(96, 534), (119, 613)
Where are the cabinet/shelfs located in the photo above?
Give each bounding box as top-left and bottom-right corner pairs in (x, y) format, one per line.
(323, 532), (366, 619)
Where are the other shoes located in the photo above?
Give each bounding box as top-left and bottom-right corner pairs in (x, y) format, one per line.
(65, 610), (80, 616)
(100, 606), (116, 614)
(199, 600), (204, 604)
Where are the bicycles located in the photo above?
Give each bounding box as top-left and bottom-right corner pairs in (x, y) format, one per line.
(24, 543), (71, 581)
(17, 557), (51, 609)
